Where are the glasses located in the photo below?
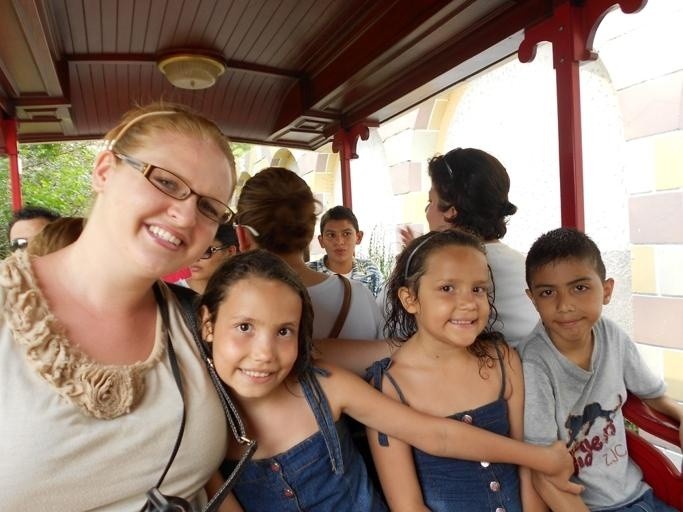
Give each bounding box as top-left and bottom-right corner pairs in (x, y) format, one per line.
(200, 245), (230, 259)
(9, 238), (27, 251)
(114, 151), (234, 226)
(431, 153), (453, 180)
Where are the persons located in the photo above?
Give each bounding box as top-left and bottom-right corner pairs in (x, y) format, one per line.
(375, 147), (541, 349)
(0, 93), (406, 512)
(172, 167), (387, 340)
(364, 229), (549, 512)
(513, 227), (683, 512)
(192, 248), (586, 512)
(25, 217), (88, 257)
(7, 205), (62, 254)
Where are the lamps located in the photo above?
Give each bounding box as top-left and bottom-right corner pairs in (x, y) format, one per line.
(156, 50), (225, 91)
